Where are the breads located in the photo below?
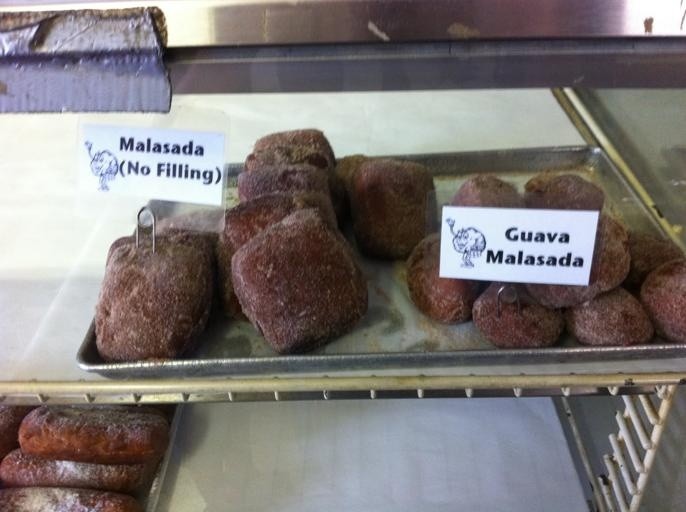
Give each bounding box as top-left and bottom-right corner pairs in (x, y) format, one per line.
(0, 400), (169, 512)
(90, 127), (686, 361)
(1, 7), (173, 115)
